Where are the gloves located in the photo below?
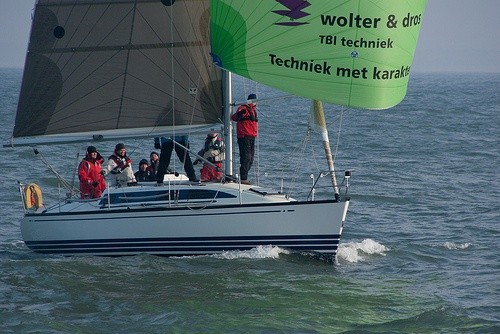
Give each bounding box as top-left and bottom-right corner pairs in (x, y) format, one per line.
(240, 109), (246, 114)
(101, 169), (106, 174)
(92, 180), (99, 186)
(155, 143), (161, 148)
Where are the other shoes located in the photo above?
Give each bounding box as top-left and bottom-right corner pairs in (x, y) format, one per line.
(157, 180), (163, 184)
(241, 180), (252, 185)
(189, 178), (200, 182)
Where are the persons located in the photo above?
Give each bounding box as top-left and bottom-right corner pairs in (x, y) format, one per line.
(134, 159), (149, 182)
(107, 143), (136, 186)
(78, 146), (108, 199)
(154, 99), (200, 184)
(146, 152), (171, 182)
(192, 130), (225, 180)
(231, 94), (259, 186)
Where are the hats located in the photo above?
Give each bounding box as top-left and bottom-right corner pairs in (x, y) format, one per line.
(116, 143), (124, 150)
(248, 94), (256, 100)
(87, 146), (96, 152)
(139, 159), (149, 166)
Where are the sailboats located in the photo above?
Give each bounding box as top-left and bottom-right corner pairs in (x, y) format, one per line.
(18, 0), (428, 267)
